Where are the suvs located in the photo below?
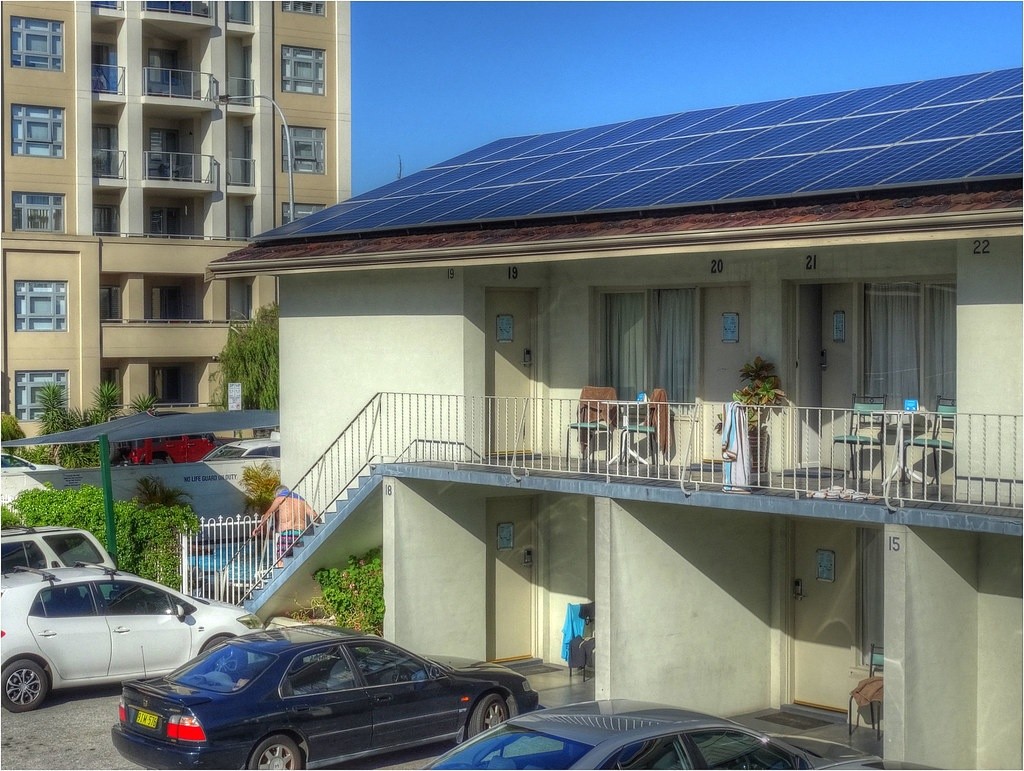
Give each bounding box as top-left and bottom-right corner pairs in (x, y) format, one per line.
(1, 526), (116, 574)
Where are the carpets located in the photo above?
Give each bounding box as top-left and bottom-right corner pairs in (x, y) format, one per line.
(511, 665), (564, 676)
(755, 712), (835, 730)
(681, 468), (722, 472)
(487, 455), (549, 461)
(777, 474), (835, 478)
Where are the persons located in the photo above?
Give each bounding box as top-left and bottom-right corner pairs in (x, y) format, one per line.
(253, 486), (323, 569)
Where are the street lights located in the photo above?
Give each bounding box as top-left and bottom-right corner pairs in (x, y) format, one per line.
(214, 94), (295, 223)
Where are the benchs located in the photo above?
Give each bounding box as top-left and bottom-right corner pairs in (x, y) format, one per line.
(201, 671), (293, 697)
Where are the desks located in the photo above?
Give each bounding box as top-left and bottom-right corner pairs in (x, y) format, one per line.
(603, 402), (650, 466)
(872, 411), (929, 485)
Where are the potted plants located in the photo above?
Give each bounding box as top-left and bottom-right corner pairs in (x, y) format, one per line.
(713, 356), (786, 473)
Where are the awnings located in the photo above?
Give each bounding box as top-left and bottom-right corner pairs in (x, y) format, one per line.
(1, 408), (280, 571)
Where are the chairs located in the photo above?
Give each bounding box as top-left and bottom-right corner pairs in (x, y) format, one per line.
(327, 660), (354, 690)
(565, 386), (668, 470)
(65, 586), (93, 616)
(562, 601), (595, 682)
(849, 643), (884, 741)
(901, 395), (957, 497)
(828, 394), (887, 487)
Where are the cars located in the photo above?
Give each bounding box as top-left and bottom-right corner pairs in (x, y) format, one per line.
(1, 453), (65, 473)
(1, 561), (266, 713)
(111, 625), (540, 770)
(123, 407), (280, 465)
(418, 699), (942, 770)
